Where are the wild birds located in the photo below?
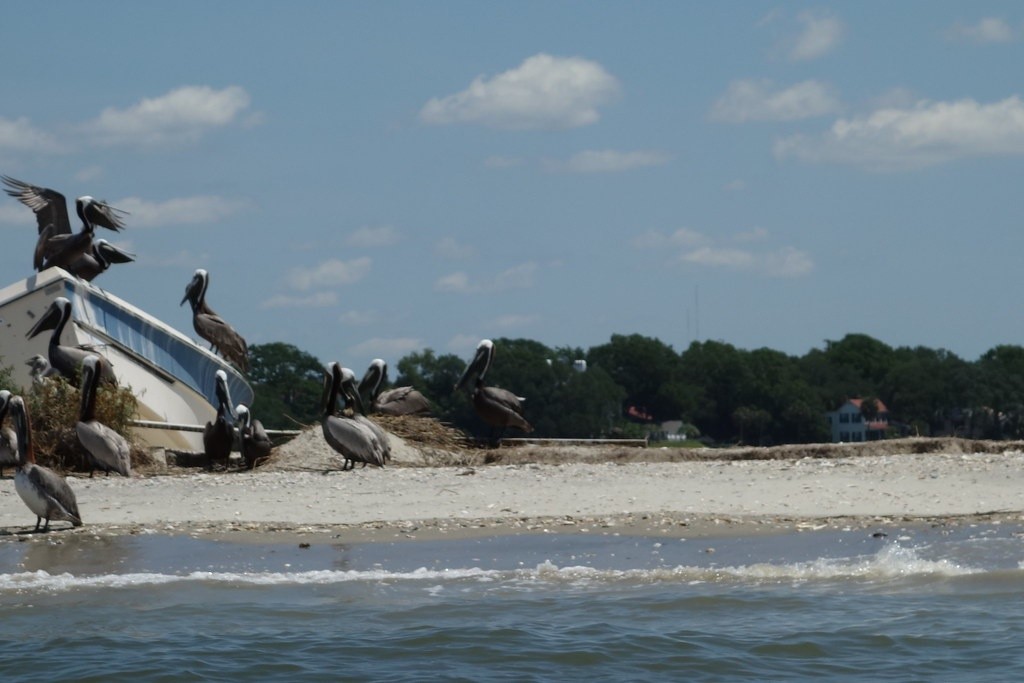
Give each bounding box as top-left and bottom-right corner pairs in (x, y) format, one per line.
(0, 173), (538, 533)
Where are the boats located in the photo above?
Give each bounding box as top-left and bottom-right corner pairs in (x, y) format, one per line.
(0, 266), (256, 466)
